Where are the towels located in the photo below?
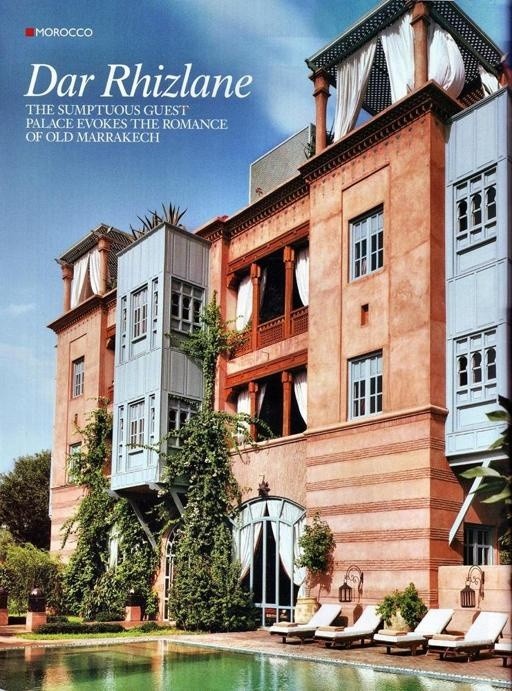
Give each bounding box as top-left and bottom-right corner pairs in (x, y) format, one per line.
(498, 638), (511, 646)
(319, 627), (344, 633)
(378, 630), (407, 637)
(433, 634), (464, 642)
(273, 622), (298, 628)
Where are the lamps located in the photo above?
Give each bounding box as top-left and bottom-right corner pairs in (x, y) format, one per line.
(339, 565), (363, 603)
(460, 566), (484, 609)
(428, 612), (509, 662)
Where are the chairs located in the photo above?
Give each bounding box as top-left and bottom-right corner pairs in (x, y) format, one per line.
(270, 605), (342, 646)
(373, 609), (454, 656)
(314, 605), (384, 649)
(490, 638), (511, 667)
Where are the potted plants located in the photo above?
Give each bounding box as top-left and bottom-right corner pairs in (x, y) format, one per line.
(375, 583), (428, 632)
